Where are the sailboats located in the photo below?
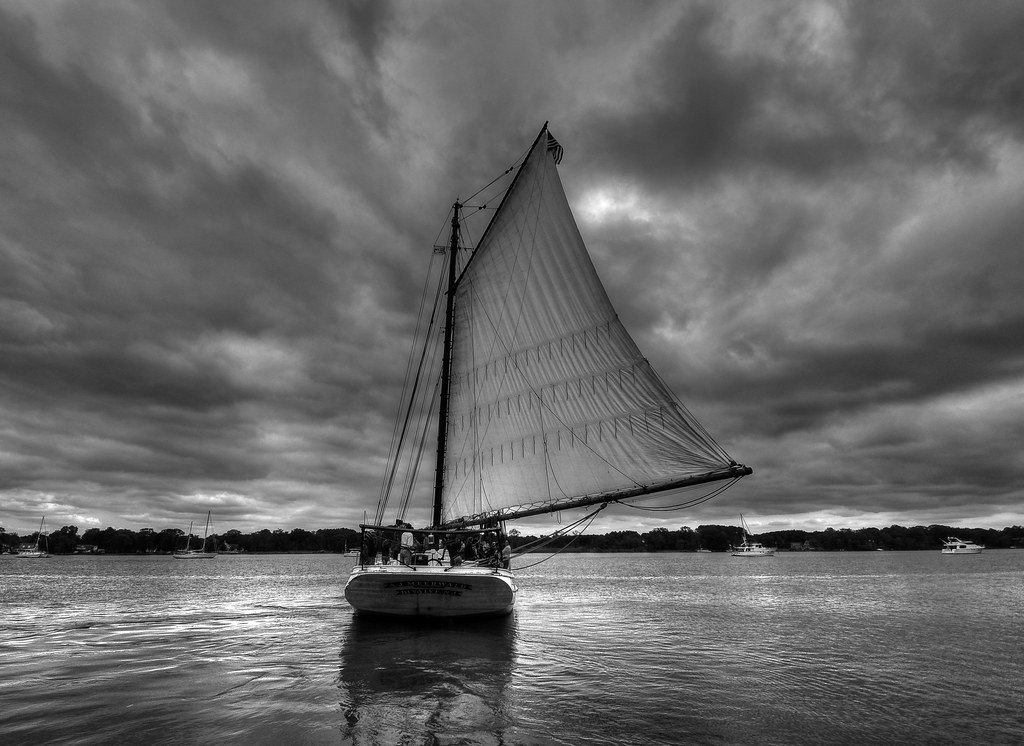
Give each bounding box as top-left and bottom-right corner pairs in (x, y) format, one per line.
(730, 512), (777, 557)
(344, 115), (753, 616)
(15, 516), (50, 558)
(173, 511), (218, 559)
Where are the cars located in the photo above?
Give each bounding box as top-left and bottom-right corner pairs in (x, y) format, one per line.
(697, 548), (713, 553)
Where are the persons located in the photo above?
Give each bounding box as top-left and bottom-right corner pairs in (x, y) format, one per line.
(361, 519), (511, 569)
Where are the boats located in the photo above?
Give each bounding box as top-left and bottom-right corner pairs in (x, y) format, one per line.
(344, 549), (360, 557)
(2, 551), (10, 555)
(942, 536), (985, 555)
(74, 544), (105, 555)
